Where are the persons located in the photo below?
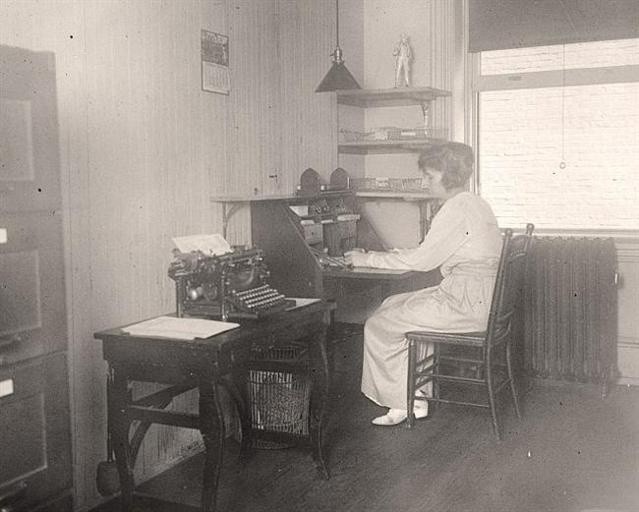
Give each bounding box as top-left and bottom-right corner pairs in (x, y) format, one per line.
(343, 143), (503, 427)
(394, 32), (412, 91)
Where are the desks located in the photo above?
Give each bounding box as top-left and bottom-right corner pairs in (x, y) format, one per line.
(244, 200), (418, 444)
(94, 298), (338, 512)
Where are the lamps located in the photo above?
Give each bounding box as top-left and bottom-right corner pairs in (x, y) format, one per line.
(315, 0), (361, 91)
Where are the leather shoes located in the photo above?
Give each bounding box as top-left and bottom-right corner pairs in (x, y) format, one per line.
(372, 409), (428, 425)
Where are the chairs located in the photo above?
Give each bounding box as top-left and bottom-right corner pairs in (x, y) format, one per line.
(406, 223), (533, 442)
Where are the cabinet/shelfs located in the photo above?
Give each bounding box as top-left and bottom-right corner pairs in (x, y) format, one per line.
(338, 88), (456, 200)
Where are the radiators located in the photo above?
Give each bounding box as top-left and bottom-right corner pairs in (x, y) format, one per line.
(440, 233), (623, 398)
